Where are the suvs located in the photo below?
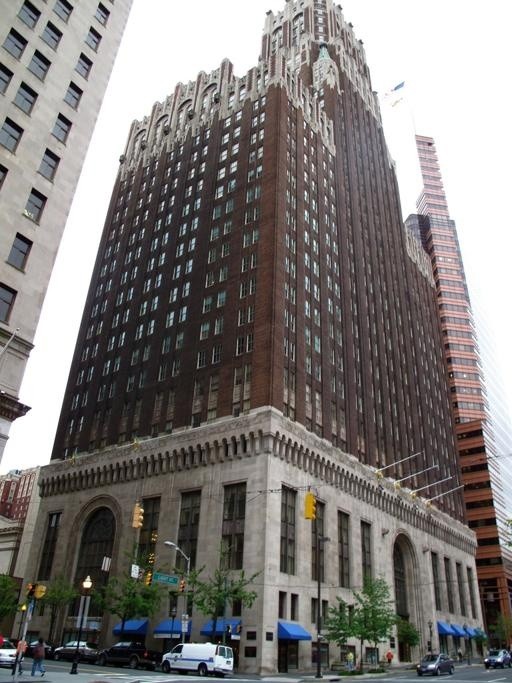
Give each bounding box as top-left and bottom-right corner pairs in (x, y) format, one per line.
(483, 648), (512, 669)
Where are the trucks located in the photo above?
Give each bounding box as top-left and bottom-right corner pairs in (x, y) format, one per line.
(160, 642), (235, 677)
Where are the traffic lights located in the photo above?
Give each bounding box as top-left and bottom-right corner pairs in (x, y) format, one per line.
(146, 571), (153, 586)
(236, 624), (243, 634)
(179, 580), (186, 593)
(132, 506), (144, 529)
(303, 492), (318, 520)
(227, 624), (231, 633)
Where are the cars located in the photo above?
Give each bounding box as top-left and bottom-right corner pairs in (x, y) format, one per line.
(0, 637), (101, 668)
(416, 653), (456, 676)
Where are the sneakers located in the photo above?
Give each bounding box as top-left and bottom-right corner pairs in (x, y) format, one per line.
(41, 672), (45, 677)
(12, 670), (23, 675)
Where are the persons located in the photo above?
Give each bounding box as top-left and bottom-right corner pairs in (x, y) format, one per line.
(346, 648), (354, 670)
(458, 646), (463, 663)
(386, 648), (394, 668)
(30, 636), (45, 676)
(11, 635), (26, 675)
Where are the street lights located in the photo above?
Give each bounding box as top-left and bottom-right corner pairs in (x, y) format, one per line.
(163, 541), (192, 645)
(69, 575), (94, 674)
(169, 606), (178, 647)
(15, 603), (28, 642)
(427, 619), (433, 653)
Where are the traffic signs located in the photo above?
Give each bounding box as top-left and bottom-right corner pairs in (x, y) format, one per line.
(153, 572), (179, 585)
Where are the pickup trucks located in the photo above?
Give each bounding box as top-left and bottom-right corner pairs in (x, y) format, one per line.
(95, 641), (162, 671)
